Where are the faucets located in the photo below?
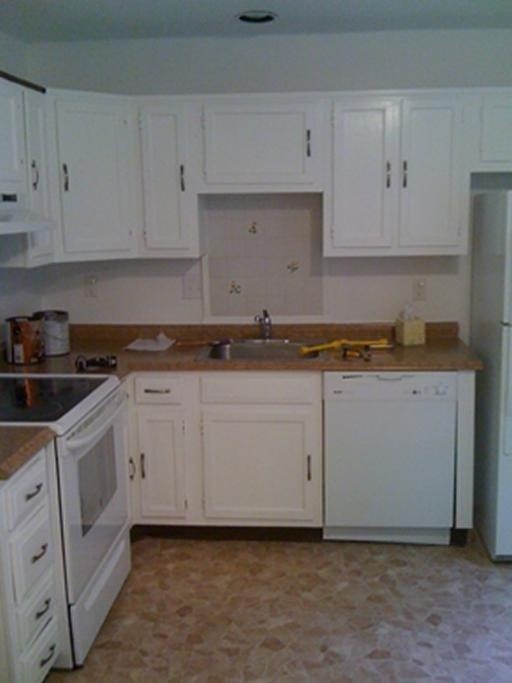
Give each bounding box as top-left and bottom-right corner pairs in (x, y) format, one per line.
(254, 309), (271, 339)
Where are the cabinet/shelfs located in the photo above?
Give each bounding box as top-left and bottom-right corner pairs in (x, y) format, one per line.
(1, 72), (195, 269)
(199, 372), (322, 528)
(0, 432), (73, 682)
(323, 92), (511, 258)
(132, 374), (197, 525)
(197, 94), (323, 193)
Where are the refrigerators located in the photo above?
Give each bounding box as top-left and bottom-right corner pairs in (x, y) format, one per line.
(477, 190), (512, 564)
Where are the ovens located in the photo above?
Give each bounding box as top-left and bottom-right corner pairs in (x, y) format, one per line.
(55, 375), (132, 667)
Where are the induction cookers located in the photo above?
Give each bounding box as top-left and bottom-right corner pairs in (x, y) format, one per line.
(1, 370), (122, 437)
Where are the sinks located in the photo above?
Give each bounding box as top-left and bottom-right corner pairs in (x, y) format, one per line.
(208, 338), (320, 363)
